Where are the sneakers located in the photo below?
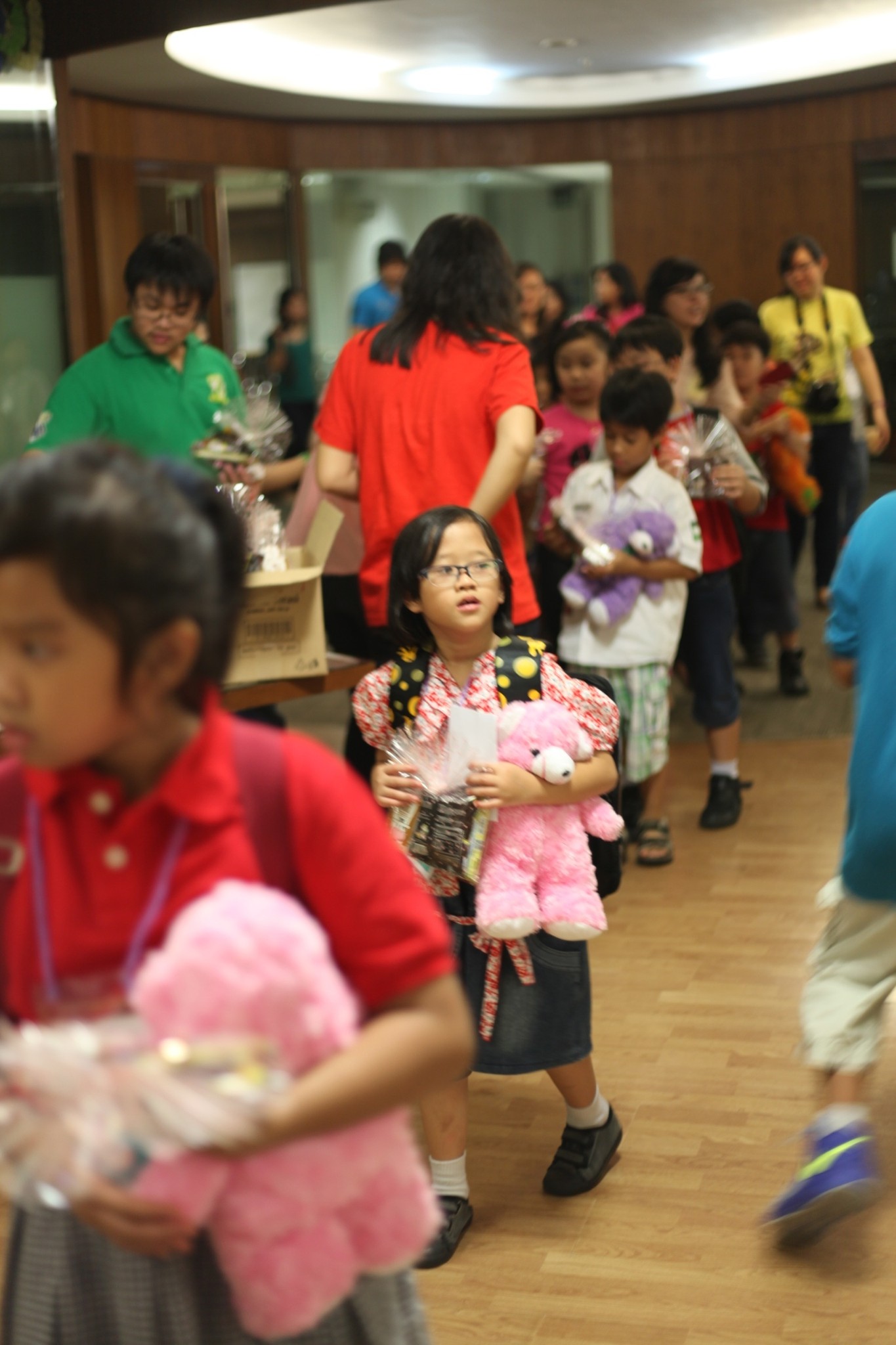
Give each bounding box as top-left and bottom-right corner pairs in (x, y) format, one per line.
(541, 1103), (623, 1196)
(411, 1195), (474, 1269)
(759, 1116), (881, 1254)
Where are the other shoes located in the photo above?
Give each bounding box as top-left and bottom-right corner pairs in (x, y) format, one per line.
(777, 647), (810, 694)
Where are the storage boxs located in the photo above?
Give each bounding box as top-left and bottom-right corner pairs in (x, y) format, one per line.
(220, 497), (346, 688)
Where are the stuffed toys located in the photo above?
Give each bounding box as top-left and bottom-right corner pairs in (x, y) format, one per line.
(472, 699), (626, 943)
(117, 878), (447, 1342)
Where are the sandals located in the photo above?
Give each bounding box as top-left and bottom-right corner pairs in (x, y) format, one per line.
(636, 820), (674, 865)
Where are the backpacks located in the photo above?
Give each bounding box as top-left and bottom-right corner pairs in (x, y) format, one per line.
(383, 633), (624, 901)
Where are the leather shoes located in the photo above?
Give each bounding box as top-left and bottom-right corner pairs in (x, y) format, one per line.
(700, 774), (752, 828)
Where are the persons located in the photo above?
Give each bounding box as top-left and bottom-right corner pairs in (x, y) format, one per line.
(541, 363), (701, 864)
(0, 433), (483, 1344)
(234, 209), (894, 700)
(583, 313), (770, 827)
(347, 498), (628, 1273)
(19, 231), (266, 498)
(753, 482), (891, 1258)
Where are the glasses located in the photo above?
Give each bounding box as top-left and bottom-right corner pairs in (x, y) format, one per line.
(137, 300), (202, 324)
(417, 558), (505, 588)
